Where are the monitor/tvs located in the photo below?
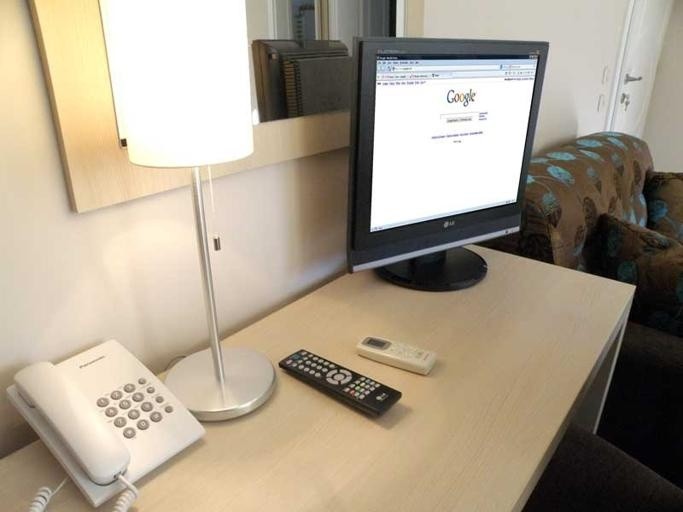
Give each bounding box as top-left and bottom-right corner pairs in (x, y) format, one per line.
(345, 35), (550, 293)
(250, 39), (352, 124)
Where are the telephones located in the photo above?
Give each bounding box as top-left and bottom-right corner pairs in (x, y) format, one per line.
(5, 339), (206, 509)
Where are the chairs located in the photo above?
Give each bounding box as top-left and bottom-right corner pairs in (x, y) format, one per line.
(508, 133), (680, 461)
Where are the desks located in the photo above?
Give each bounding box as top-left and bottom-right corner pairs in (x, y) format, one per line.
(0, 243), (638, 512)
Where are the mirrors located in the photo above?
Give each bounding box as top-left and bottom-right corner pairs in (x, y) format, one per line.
(31, 2), (398, 216)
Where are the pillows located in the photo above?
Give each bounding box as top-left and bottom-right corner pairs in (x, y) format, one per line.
(601, 172), (683, 302)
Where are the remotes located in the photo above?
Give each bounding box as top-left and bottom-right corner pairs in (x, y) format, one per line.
(357, 334), (437, 375)
(279, 348), (402, 418)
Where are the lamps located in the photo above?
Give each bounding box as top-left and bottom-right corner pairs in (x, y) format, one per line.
(98, 0), (276, 424)
